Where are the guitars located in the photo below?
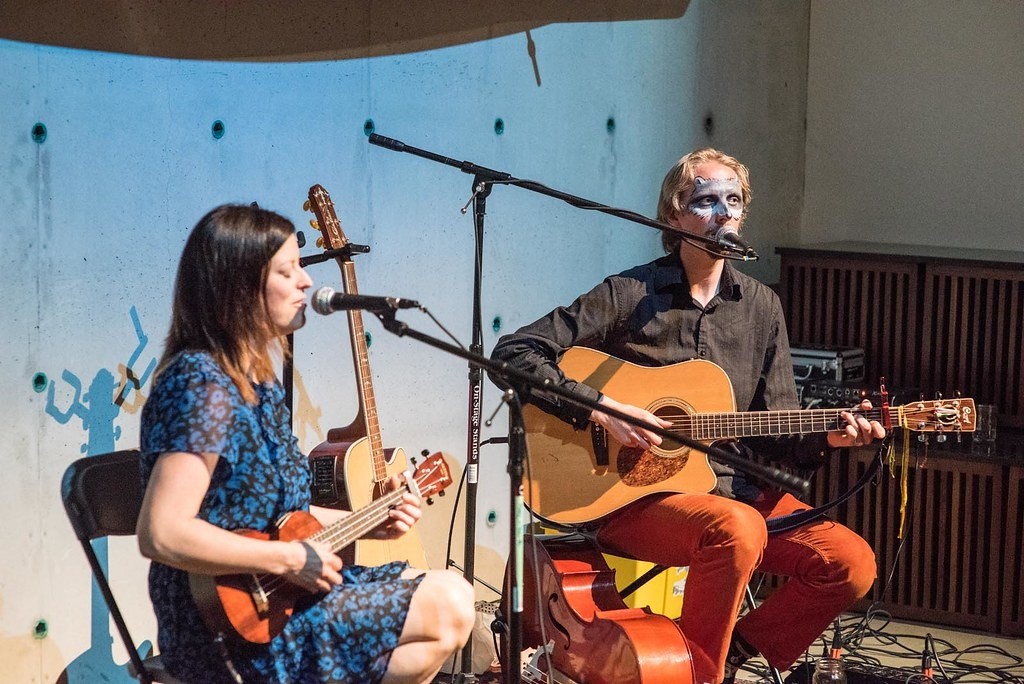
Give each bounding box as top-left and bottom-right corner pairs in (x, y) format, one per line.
(300, 181), (425, 576)
(520, 345), (977, 532)
(495, 533), (700, 684)
(187, 447), (454, 652)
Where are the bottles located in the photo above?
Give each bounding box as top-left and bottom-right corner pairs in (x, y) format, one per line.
(811, 657), (847, 684)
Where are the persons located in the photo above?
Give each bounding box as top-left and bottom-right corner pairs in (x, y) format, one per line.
(487, 146), (879, 684)
(137, 205), (478, 684)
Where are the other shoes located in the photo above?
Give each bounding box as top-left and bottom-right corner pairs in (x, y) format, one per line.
(721, 630), (759, 684)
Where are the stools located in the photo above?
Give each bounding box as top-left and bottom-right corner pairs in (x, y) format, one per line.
(540, 524), (690, 621)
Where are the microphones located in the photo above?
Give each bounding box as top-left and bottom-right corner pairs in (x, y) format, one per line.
(312, 288), (422, 316)
(714, 226), (761, 261)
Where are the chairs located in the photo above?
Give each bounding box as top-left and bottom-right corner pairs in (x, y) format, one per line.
(60, 447), (185, 684)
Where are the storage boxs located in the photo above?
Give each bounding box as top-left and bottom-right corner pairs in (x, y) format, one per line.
(760, 344), (864, 403)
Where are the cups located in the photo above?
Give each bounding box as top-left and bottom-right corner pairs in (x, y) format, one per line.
(971, 442), (997, 457)
(972, 404), (998, 442)
